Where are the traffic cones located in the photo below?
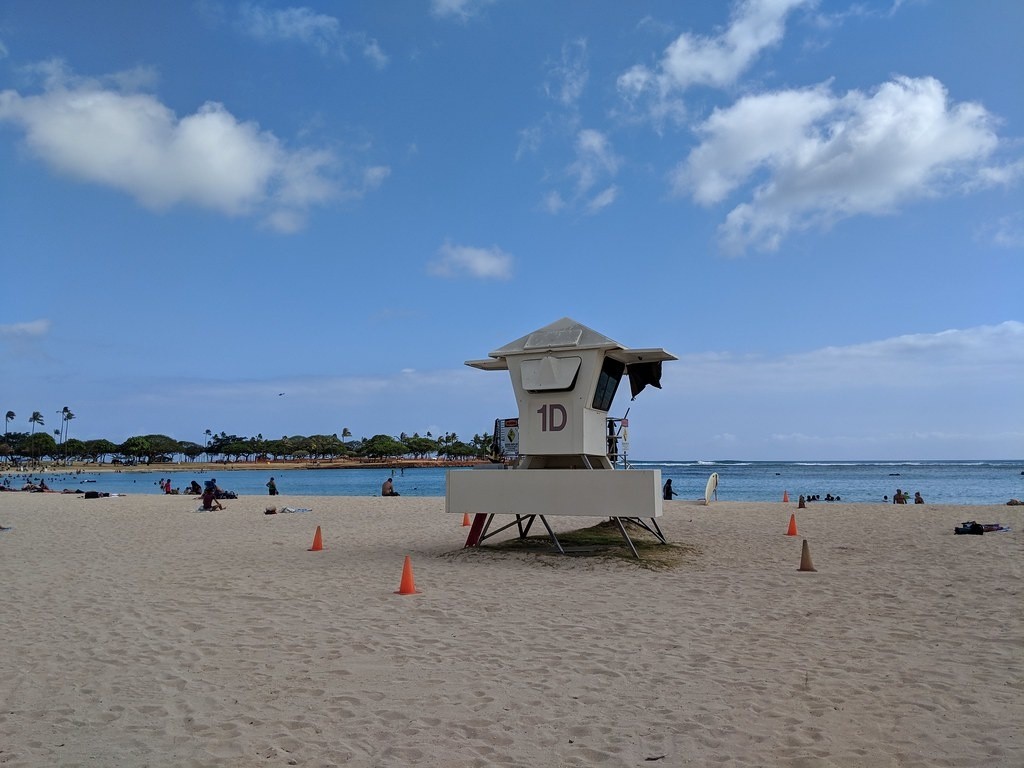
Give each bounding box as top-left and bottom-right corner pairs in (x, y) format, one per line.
(392, 556), (423, 597)
(462, 511), (471, 528)
(782, 513), (801, 536)
(781, 490), (789, 503)
(796, 539), (818, 573)
(307, 526), (327, 551)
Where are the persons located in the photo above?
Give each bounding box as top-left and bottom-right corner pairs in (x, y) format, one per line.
(202, 488), (221, 511)
(381, 478), (400, 496)
(177, 487), (181, 494)
(23, 481), (32, 489)
(893, 489), (908, 504)
(41, 479), (49, 489)
(663, 479), (678, 500)
(160, 478), (171, 494)
(4, 478), (10, 487)
(265, 477), (278, 496)
(211, 478), (223, 492)
(184, 481), (202, 495)
(914, 492), (924, 504)
(401, 465), (405, 474)
(884, 496), (887, 500)
(806, 493), (841, 502)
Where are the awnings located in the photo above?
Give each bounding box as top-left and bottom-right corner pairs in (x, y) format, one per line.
(464, 358), (508, 371)
(606, 347), (680, 364)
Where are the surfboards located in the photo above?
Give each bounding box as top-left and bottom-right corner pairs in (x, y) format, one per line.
(703, 471), (720, 506)
